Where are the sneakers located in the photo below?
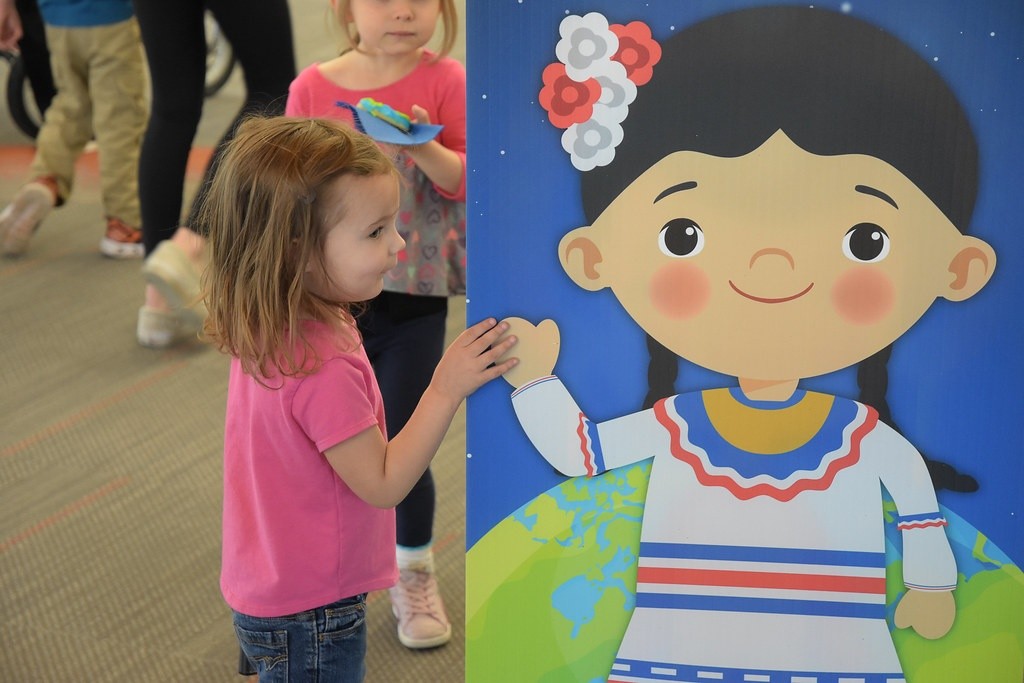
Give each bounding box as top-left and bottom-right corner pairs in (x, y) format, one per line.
(141, 241), (211, 327)
(99, 216), (148, 259)
(392, 566), (452, 649)
(139, 307), (206, 346)
(0, 179), (58, 259)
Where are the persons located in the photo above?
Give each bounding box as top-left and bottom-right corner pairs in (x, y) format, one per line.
(285, 0), (466, 649)
(0, 0), (150, 257)
(134, 0), (297, 346)
(199, 116), (520, 683)
